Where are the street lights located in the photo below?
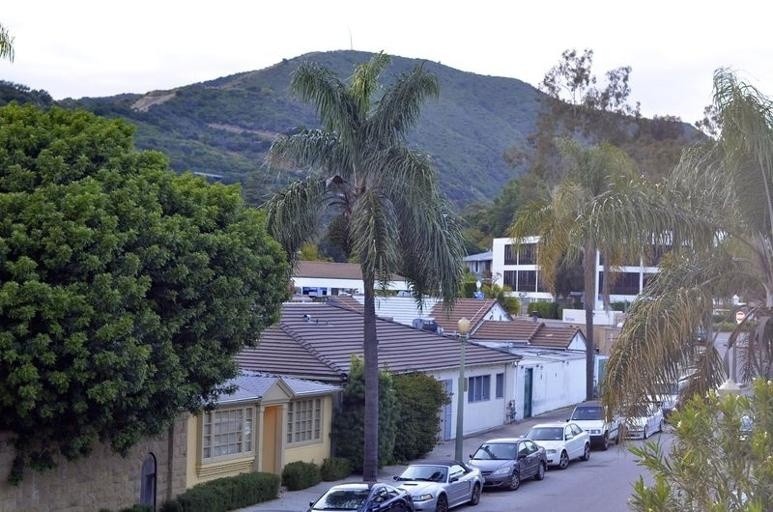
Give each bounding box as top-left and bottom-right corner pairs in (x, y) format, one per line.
(455, 317), (470, 461)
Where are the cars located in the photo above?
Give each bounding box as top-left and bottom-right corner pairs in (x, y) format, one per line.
(737, 416), (756, 443)
(729, 491), (754, 511)
